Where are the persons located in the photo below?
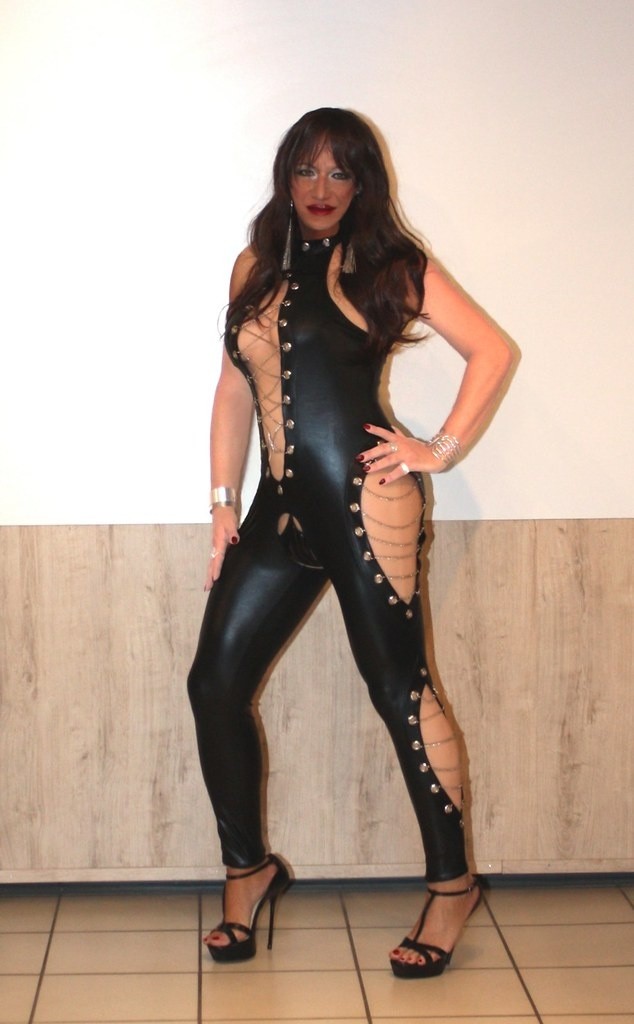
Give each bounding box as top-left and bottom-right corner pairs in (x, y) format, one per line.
(187, 107), (513, 978)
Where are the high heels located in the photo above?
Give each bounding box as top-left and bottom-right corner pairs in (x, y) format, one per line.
(389, 880), (484, 980)
(205, 855), (290, 963)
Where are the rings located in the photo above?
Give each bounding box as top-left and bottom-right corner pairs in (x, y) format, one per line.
(389, 443), (399, 452)
(208, 547), (218, 559)
(401, 462), (409, 475)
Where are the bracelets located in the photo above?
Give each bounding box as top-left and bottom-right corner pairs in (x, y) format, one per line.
(426, 427), (461, 468)
(208, 486), (242, 518)
